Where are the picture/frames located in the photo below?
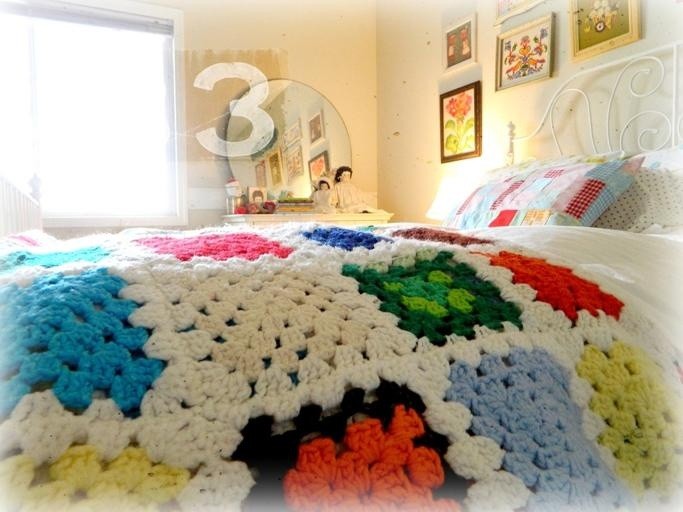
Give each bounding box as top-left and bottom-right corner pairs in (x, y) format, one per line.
(251, 160), (266, 190)
(264, 147), (283, 189)
(494, 10), (555, 94)
(304, 109), (329, 149)
(435, 78), (484, 164)
(493, 1), (547, 27)
(282, 145), (304, 186)
(441, 11), (479, 74)
(281, 117), (304, 152)
(569, 1), (643, 64)
(306, 149), (333, 193)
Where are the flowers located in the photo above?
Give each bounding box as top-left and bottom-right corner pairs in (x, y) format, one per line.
(442, 89), (476, 156)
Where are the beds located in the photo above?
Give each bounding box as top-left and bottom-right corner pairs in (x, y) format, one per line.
(0, 41), (683, 510)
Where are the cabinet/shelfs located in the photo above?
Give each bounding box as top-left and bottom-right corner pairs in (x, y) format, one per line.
(217, 212), (393, 227)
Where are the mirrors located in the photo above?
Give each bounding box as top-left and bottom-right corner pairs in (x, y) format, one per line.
(224, 77), (353, 201)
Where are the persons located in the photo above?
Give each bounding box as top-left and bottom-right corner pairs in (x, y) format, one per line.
(314, 178), (336, 214)
(333, 165), (365, 213)
(252, 190), (264, 206)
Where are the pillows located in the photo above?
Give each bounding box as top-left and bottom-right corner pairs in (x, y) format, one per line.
(447, 154), (649, 232)
(592, 167), (682, 232)
(500, 149), (624, 173)
